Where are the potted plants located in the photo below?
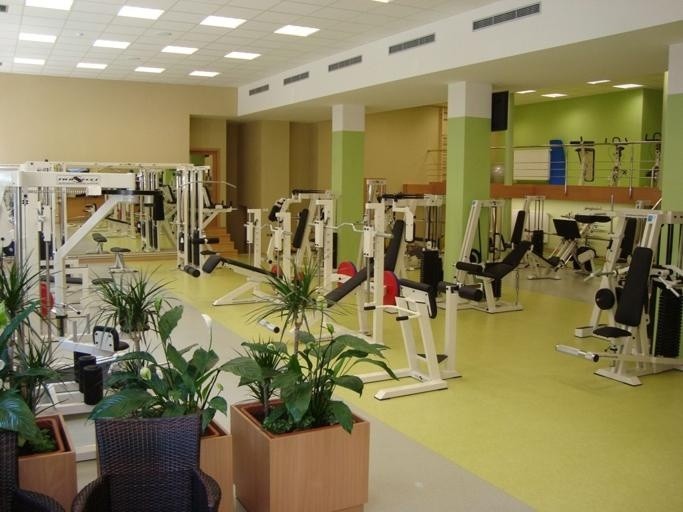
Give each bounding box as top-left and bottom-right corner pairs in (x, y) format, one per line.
(73, 300), (264, 512)
(80, 263), (237, 512)
(0, 250), (77, 512)
(227, 245), (402, 512)
(0, 295), (44, 510)
